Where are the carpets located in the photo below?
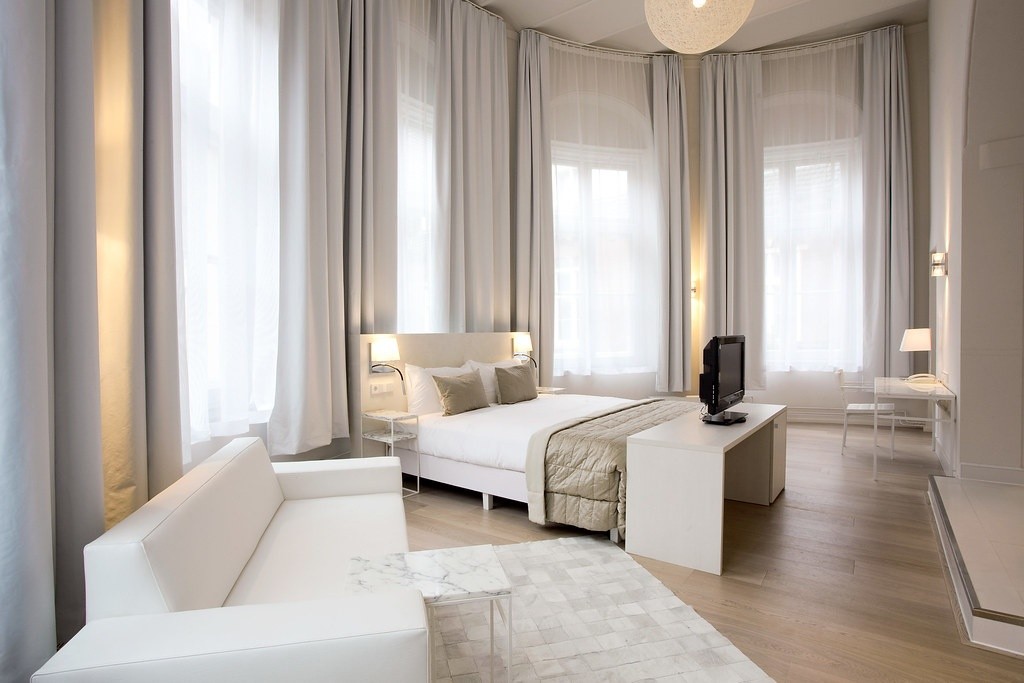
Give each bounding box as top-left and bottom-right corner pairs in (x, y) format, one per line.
(412, 532), (776, 683)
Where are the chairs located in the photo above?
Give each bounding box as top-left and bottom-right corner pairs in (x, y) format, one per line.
(837, 368), (897, 461)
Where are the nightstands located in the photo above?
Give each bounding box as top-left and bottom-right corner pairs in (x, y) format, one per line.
(536, 386), (567, 394)
(361, 409), (419, 498)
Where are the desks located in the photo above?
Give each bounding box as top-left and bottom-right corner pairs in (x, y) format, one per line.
(627, 403), (788, 575)
(406, 544), (512, 683)
(874, 376), (956, 482)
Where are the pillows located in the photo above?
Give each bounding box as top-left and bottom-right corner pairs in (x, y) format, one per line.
(469, 356), (523, 403)
(431, 368), (491, 418)
(492, 358), (539, 403)
(404, 360), (474, 415)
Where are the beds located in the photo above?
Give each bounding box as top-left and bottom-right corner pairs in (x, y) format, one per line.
(350, 331), (703, 545)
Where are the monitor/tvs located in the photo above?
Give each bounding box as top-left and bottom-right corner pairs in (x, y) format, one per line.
(699, 336), (749, 426)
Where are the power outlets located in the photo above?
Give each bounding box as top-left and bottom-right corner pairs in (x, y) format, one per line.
(940, 372), (949, 387)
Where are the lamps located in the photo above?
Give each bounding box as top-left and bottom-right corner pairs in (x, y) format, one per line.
(932, 252), (948, 276)
(511, 332), (533, 356)
(899, 328), (930, 352)
(370, 333), (400, 373)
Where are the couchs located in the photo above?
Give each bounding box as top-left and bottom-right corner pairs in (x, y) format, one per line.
(29, 436), (428, 683)
